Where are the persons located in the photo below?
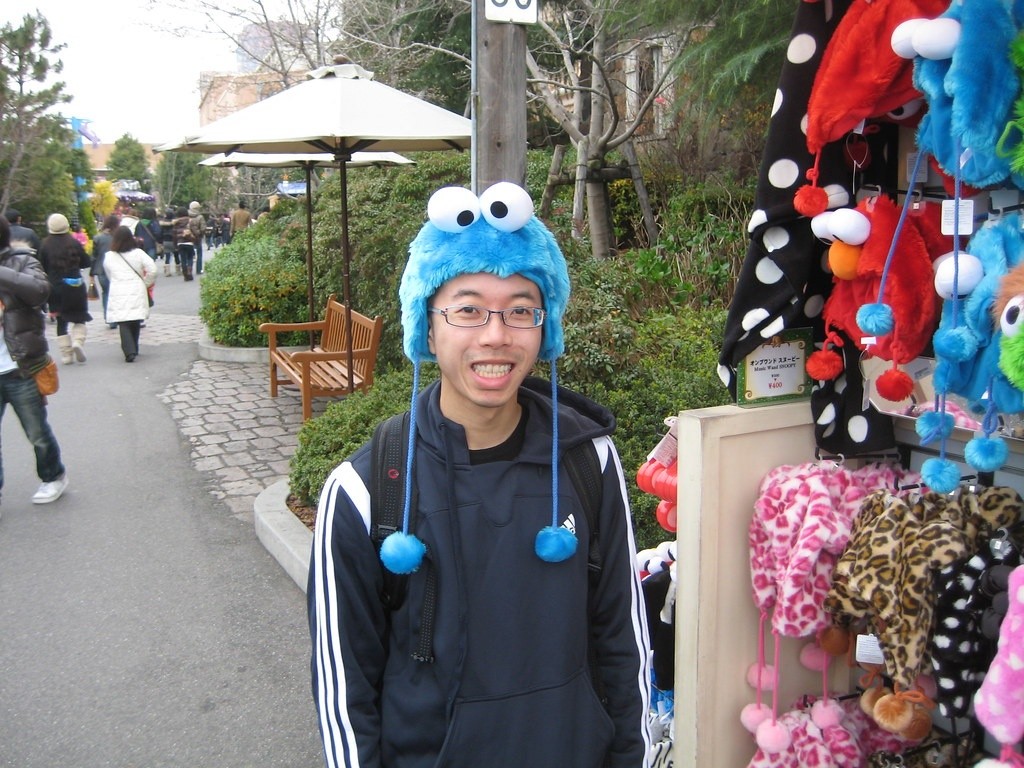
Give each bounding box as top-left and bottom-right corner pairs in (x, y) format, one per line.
(159, 208), (182, 277)
(229, 200), (253, 237)
(306, 182), (654, 768)
(187, 201), (208, 274)
(206, 214), (231, 251)
(134, 208), (160, 298)
(37, 213), (93, 364)
(103, 226), (157, 362)
(90, 215), (120, 328)
(0, 214), (69, 503)
(4, 209), (39, 250)
(120, 209), (139, 235)
(173, 208), (198, 282)
(258, 207), (270, 220)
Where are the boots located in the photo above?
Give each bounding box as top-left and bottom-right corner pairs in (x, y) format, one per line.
(176, 263), (182, 275)
(164, 264), (171, 277)
(54, 334), (74, 364)
(72, 323), (86, 362)
(183, 266), (193, 280)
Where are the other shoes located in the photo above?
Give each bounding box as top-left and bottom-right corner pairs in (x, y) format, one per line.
(126, 353), (134, 362)
(140, 322), (146, 328)
(110, 323), (117, 328)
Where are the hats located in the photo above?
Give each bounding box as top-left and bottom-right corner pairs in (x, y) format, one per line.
(379, 181), (579, 575)
(751, 3), (1023, 768)
(190, 201), (203, 211)
(48, 214), (70, 234)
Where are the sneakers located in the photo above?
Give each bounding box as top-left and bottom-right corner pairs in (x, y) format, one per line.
(31, 471), (68, 504)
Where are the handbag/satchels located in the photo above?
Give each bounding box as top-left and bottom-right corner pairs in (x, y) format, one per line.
(155, 243), (164, 255)
(32, 356), (59, 395)
(61, 277), (87, 311)
(182, 219), (195, 241)
(149, 294), (154, 307)
(87, 275), (98, 298)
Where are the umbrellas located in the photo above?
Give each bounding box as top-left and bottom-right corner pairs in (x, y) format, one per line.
(154, 55), (472, 395)
(198, 152), (417, 350)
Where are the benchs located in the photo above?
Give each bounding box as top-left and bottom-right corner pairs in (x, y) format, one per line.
(260, 294), (383, 421)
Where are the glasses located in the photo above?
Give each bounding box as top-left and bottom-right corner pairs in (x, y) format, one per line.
(427, 304), (546, 329)
